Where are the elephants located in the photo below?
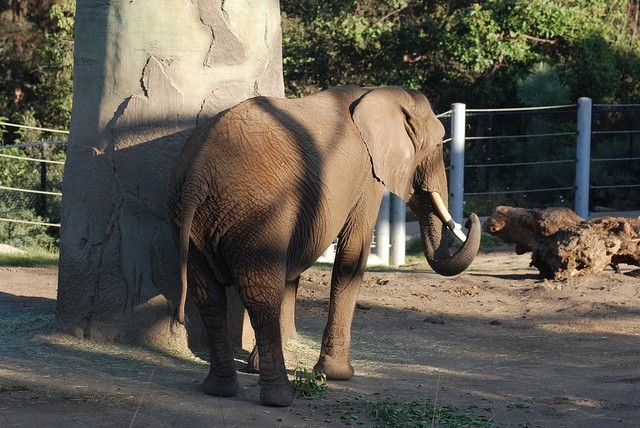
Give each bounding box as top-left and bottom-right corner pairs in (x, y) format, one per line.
(164, 84), (481, 407)
